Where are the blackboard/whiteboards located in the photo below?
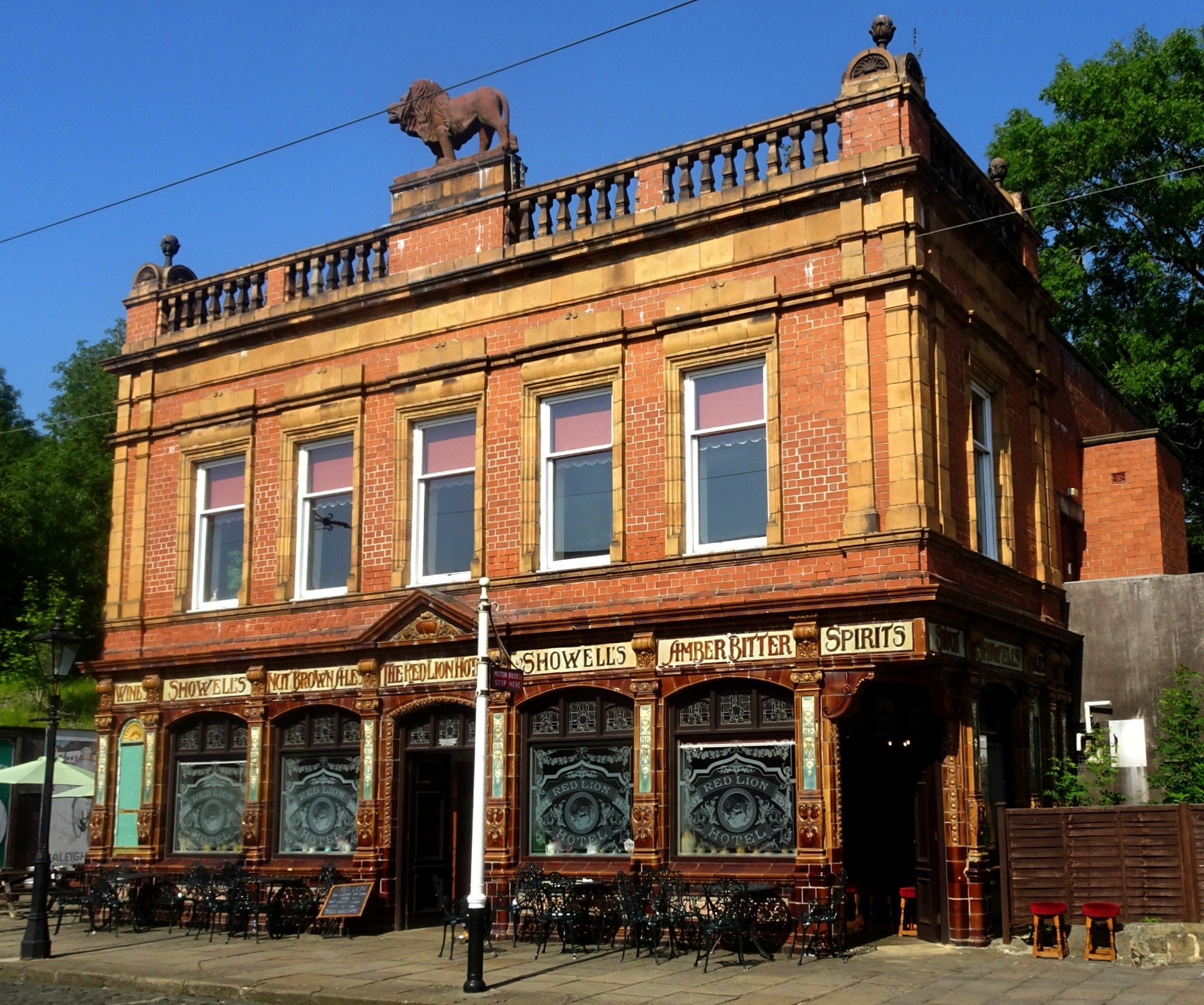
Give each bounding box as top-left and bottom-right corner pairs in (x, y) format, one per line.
(317, 882), (374, 918)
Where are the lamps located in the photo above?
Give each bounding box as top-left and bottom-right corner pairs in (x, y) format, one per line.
(1067, 488), (1079, 498)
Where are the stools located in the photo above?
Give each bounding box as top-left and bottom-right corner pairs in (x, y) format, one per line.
(1029, 902), (1069, 961)
(1082, 902), (1119, 963)
(898, 887), (918, 939)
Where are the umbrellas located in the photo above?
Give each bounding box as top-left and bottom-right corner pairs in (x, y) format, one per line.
(0, 756), (95, 841)
(51, 785), (94, 799)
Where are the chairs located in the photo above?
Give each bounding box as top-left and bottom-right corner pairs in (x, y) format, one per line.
(430, 862), (861, 966)
(13, 860), (349, 941)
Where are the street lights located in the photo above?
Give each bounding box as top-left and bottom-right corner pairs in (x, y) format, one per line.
(18, 610), (86, 960)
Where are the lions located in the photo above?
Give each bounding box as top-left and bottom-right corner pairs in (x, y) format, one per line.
(387, 80), (520, 163)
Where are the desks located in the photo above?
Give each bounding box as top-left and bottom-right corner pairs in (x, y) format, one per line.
(689, 881), (779, 968)
(83, 873), (155, 938)
(221, 875), (297, 943)
(534, 878), (618, 956)
